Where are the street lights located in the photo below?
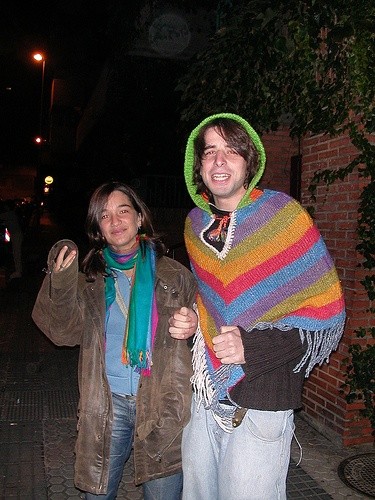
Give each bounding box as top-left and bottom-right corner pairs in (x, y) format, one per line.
(35, 136), (46, 177)
(33, 51), (46, 239)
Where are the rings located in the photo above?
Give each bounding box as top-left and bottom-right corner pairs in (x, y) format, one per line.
(60, 264), (64, 269)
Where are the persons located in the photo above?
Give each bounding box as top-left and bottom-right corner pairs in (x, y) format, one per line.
(31, 182), (199, 500)
(165, 113), (346, 500)
